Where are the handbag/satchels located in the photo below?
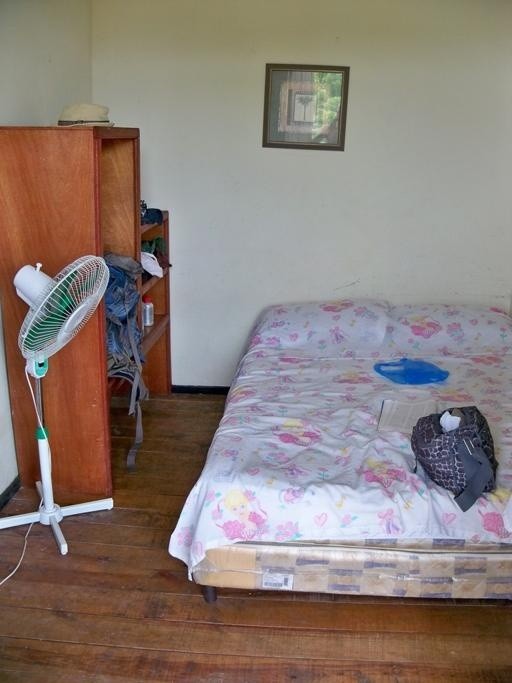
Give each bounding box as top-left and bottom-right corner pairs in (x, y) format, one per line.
(411, 405), (498, 510)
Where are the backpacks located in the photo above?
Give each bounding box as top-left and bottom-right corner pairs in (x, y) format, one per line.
(103, 262), (149, 474)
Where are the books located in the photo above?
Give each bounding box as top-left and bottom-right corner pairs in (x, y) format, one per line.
(376, 400), (478, 434)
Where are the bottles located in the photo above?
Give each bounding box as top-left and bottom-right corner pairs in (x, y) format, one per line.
(142, 295), (155, 326)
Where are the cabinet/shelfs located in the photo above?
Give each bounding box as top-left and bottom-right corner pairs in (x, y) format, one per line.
(0, 126), (169, 498)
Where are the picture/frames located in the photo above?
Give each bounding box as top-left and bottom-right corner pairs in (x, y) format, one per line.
(263, 64), (350, 150)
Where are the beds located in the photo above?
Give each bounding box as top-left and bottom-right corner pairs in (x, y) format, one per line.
(190, 299), (512, 603)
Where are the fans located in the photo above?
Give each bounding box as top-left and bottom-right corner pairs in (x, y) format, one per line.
(0, 252), (113, 558)
